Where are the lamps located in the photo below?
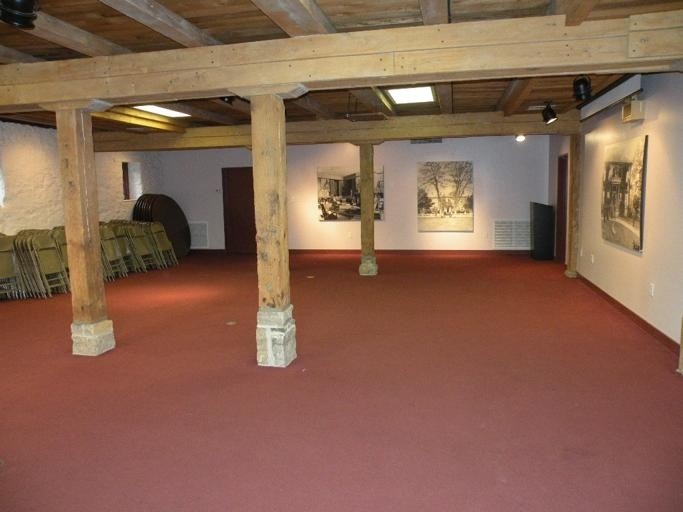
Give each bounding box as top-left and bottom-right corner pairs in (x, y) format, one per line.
(0, 0), (38, 30)
(573, 74), (593, 100)
(542, 101), (558, 125)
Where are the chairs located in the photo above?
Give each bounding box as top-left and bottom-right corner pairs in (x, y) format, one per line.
(0, 219), (180, 300)
